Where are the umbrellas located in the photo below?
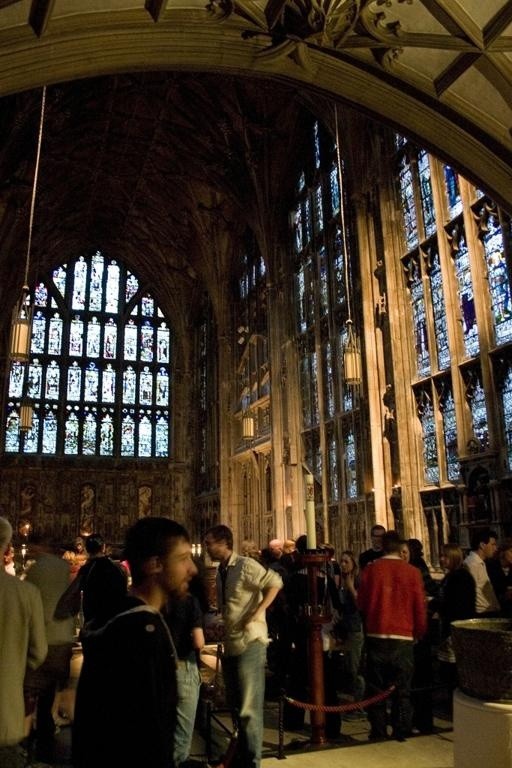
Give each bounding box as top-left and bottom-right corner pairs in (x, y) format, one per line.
(281, 694), (452, 741)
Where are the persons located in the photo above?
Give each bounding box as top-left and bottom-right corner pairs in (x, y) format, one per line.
(240, 535), (368, 744)
(202, 525), (284, 765)
(356, 525), (509, 740)
(1, 516), (207, 767)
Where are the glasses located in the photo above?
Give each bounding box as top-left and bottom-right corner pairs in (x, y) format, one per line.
(333, 102), (363, 384)
(242, 405), (254, 441)
(18, 395), (35, 431)
(8, 85), (47, 363)
